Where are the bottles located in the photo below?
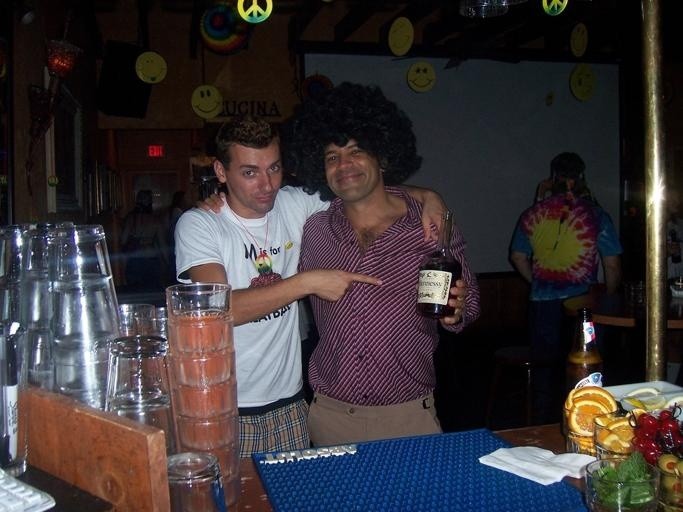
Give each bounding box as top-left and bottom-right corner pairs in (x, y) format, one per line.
(560, 308), (604, 439)
(415, 209), (463, 322)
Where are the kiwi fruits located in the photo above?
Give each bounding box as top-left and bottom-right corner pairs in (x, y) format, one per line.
(657, 454), (683, 505)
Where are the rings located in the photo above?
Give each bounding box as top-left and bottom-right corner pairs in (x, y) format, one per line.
(444, 211), (451, 221)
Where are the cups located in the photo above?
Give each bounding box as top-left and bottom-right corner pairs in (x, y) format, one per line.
(562, 417), (683, 511)
(1, 219), (245, 510)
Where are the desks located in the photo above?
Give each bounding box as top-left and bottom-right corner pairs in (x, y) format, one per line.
(561, 294), (682, 354)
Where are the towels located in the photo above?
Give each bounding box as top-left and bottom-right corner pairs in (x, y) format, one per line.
(475, 444), (595, 488)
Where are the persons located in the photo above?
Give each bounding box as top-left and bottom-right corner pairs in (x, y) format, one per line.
(161, 191), (188, 247)
(120, 189), (170, 291)
(195, 81), (480, 450)
(172, 111), (457, 461)
(664, 152), (682, 386)
(509, 151), (623, 428)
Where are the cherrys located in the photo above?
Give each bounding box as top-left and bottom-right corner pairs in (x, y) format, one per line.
(628, 403), (683, 466)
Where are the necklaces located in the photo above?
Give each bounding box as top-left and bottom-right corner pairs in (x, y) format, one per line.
(227, 204), (274, 277)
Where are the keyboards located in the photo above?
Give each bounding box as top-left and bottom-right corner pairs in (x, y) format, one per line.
(0, 468), (56, 512)
